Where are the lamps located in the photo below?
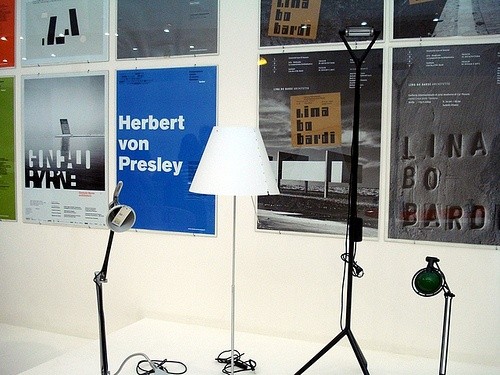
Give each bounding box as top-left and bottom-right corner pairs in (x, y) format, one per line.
(411, 255), (458, 375)
(187, 126), (280, 374)
(90, 181), (139, 375)
(295, 22), (386, 375)
